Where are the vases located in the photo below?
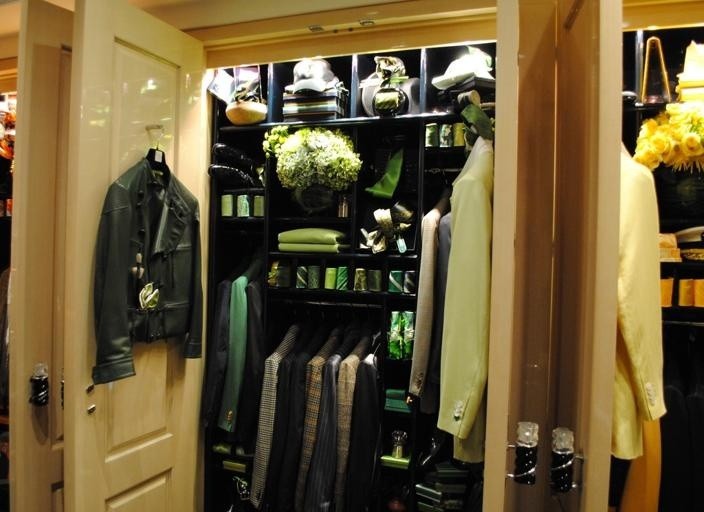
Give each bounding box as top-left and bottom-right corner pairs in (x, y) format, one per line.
(295, 187), (334, 212)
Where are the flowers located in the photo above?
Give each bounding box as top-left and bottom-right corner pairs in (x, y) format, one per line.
(261, 123), (360, 187)
(630, 103), (703, 171)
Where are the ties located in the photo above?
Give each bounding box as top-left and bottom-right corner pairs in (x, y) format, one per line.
(460, 103), (492, 147)
(369, 270), (381, 292)
(388, 271), (403, 293)
(276, 266), (290, 288)
(324, 268), (336, 289)
(354, 269), (368, 291)
(365, 147), (404, 198)
(295, 266), (307, 288)
(404, 271), (415, 293)
(336, 267), (348, 291)
(308, 266), (320, 289)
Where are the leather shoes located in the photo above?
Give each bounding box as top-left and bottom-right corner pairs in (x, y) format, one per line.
(212, 143), (263, 188)
(208, 164), (262, 190)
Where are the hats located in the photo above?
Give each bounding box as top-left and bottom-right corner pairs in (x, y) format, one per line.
(293, 59), (334, 95)
(432, 45), (495, 92)
(359, 55), (421, 115)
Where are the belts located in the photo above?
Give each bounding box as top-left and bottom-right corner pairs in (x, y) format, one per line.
(416, 431), (449, 467)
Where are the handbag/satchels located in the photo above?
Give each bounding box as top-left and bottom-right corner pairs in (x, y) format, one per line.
(638, 36), (672, 105)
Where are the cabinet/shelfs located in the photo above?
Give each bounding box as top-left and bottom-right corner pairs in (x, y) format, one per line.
(199, 23), (496, 512)
(620, 1), (704, 512)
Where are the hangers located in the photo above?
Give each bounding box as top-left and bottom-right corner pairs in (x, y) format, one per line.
(294, 299), (383, 332)
(424, 167), (449, 189)
(144, 124), (166, 166)
